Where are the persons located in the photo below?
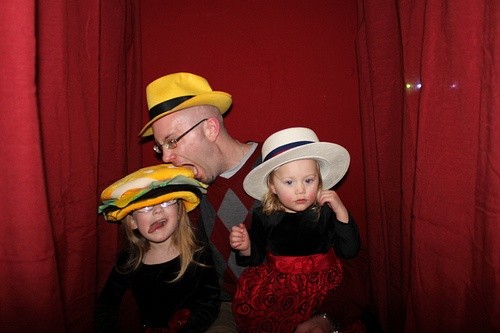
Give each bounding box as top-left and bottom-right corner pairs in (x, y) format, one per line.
(90, 164), (220, 332)
(228, 127), (359, 333)
(137, 72), (375, 333)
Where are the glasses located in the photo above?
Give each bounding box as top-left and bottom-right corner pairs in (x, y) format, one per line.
(136, 199), (177, 212)
(153, 119), (208, 153)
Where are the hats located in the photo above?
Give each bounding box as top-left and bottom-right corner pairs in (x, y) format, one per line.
(98, 164), (209, 221)
(243, 127), (350, 201)
(139, 72), (232, 137)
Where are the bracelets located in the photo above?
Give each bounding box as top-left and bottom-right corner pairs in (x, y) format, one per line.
(323, 311), (339, 332)
